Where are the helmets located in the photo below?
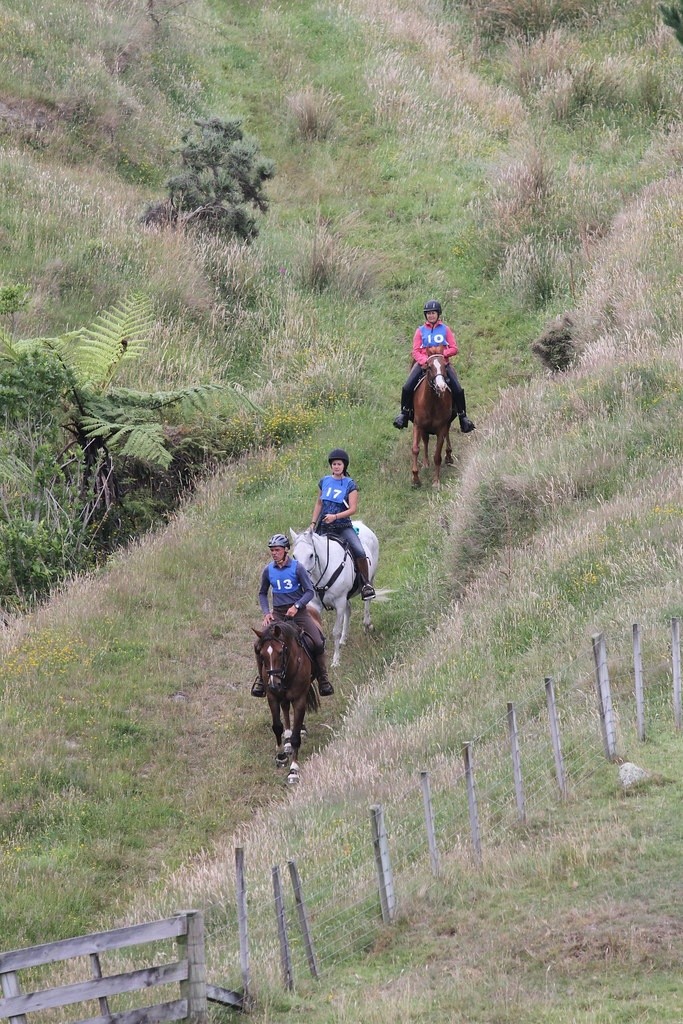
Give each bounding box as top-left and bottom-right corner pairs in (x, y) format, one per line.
(268, 534), (290, 550)
(328, 448), (349, 466)
(423, 300), (442, 315)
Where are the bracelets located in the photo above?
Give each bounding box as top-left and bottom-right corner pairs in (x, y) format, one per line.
(311, 522), (316, 525)
(295, 604), (299, 609)
(336, 515), (337, 520)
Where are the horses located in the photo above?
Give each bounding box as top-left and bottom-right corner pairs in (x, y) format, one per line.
(410, 344), (458, 489)
(290, 519), (380, 669)
(252, 606), (325, 783)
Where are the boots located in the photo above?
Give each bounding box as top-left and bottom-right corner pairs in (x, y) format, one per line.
(452, 389), (475, 433)
(251, 654), (265, 697)
(313, 651), (332, 695)
(393, 390), (413, 429)
(355, 557), (375, 596)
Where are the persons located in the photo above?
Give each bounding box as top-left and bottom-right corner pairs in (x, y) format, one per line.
(393, 301), (471, 433)
(253, 534), (332, 695)
(307, 449), (374, 597)
